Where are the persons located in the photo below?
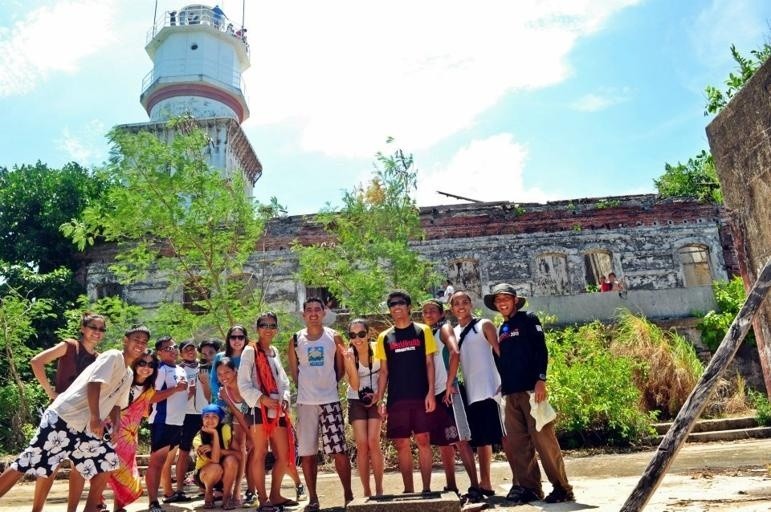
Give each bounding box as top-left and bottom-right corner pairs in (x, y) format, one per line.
(169, 4), (248, 52)
(608, 272), (624, 289)
(1, 313), (302, 512)
(289, 280), (575, 511)
(597, 276), (612, 292)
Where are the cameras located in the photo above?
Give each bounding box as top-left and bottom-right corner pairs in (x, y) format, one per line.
(357, 384), (375, 409)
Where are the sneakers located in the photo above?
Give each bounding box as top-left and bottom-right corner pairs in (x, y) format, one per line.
(443, 486), (575, 504)
(162, 490), (191, 503)
(296, 484), (307, 501)
(149, 500), (165, 512)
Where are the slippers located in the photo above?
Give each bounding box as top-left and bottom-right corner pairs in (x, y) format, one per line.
(304, 503), (319, 512)
(198, 488), (299, 512)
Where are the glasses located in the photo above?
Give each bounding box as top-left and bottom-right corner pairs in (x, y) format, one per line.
(349, 331), (368, 338)
(87, 325), (106, 332)
(259, 324), (276, 329)
(138, 360), (154, 368)
(230, 335), (245, 340)
(160, 344), (179, 352)
(388, 301), (406, 306)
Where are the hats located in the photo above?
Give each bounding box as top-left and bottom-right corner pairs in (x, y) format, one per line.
(179, 341), (194, 351)
(202, 404), (224, 421)
(485, 283), (525, 311)
